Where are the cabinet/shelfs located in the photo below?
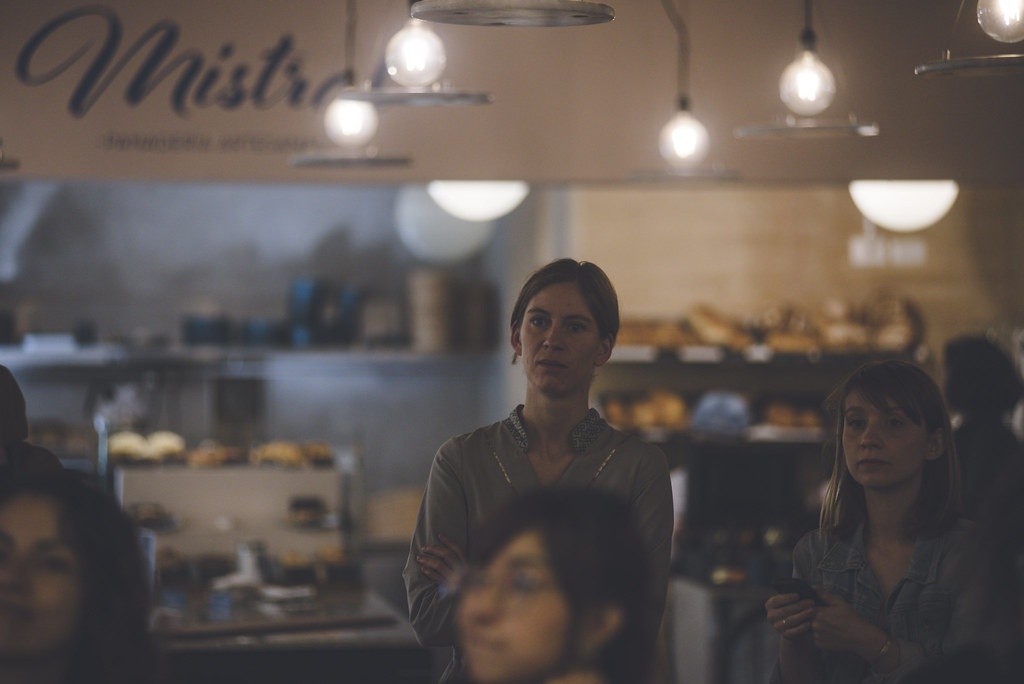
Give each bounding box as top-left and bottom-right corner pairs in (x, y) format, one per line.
(589, 343), (936, 451)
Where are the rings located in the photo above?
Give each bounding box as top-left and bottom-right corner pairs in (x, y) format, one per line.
(782, 618), (788, 626)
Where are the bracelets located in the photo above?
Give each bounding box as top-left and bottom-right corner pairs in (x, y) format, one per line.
(870, 639), (891, 664)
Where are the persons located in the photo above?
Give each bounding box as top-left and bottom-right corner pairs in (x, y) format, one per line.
(674, 335), (1024, 644)
(453, 481), (659, 684)
(0, 364), (153, 684)
(764, 361), (1018, 683)
(401, 259), (674, 684)
